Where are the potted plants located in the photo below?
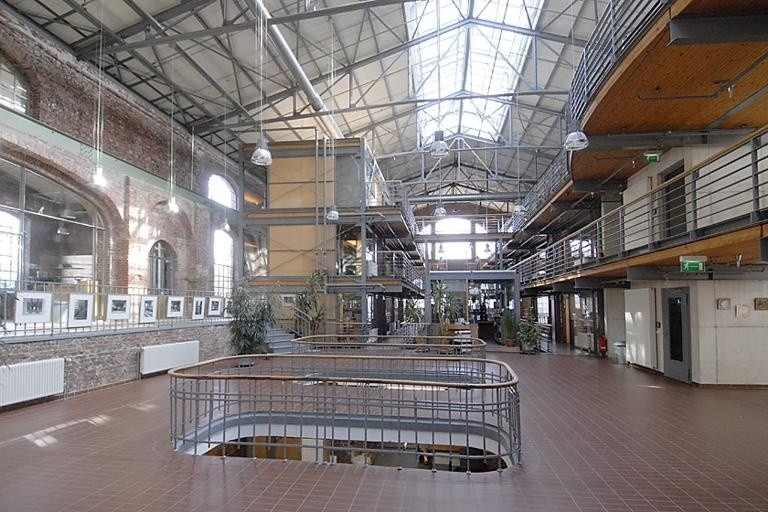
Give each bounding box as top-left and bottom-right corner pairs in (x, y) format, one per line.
(222, 269), (539, 366)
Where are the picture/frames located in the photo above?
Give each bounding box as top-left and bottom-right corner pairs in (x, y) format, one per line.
(15, 291), (235, 328)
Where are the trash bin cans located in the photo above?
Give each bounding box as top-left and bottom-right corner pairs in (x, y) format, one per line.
(613, 341), (626, 365)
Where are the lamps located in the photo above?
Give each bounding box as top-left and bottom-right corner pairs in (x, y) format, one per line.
(562, 1), (590, 151)
(429, 2), (451, 158)
(326, 18), (340, 220)
(433, 158), (447, 217)
(483, 168), (492, 252)
(511, 83), (528, 216)
(163, 42), (179, 213)
(250, 0), (272, 166)
(437, 228), (446, 252)
(86, 1), (109, 189)
(219, 94), (233, 233)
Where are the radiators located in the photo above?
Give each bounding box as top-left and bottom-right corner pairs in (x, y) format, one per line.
(577, 332), (595, 353)
(0, 340), (200, 407)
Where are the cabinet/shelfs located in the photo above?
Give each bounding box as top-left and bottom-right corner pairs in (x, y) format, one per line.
(52, 255), (93, 284)
